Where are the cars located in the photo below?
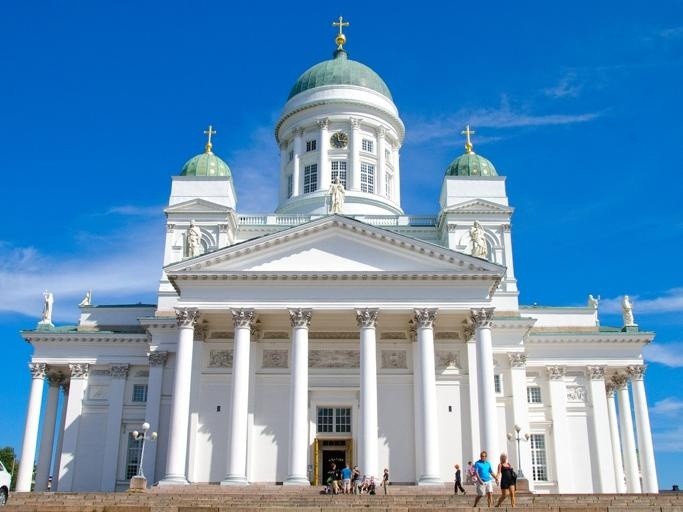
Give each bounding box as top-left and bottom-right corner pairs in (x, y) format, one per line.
(0, 462), (12, 508)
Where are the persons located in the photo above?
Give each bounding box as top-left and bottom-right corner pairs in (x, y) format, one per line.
(186, 220), (201, 257)
(473, 451), (499, 507)
(587, 294), (599, 322)
(454, 464), (466, 495)
(42, 293), (53, 321)
(326, 463), (377, 495)
(465, 461), (473, 485)
(380, 469), (389, 494)
(494, 454), (515, 508)
(469, 220), (487, 258)
(328, 176), (345, 213)
(622, 296), (633, 325)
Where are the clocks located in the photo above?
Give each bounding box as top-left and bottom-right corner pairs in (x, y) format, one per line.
(330, 131), (348, 149)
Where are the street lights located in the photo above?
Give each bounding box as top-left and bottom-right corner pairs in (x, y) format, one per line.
(506, 425), (530, 479)
(133, 423), (157, 477)
